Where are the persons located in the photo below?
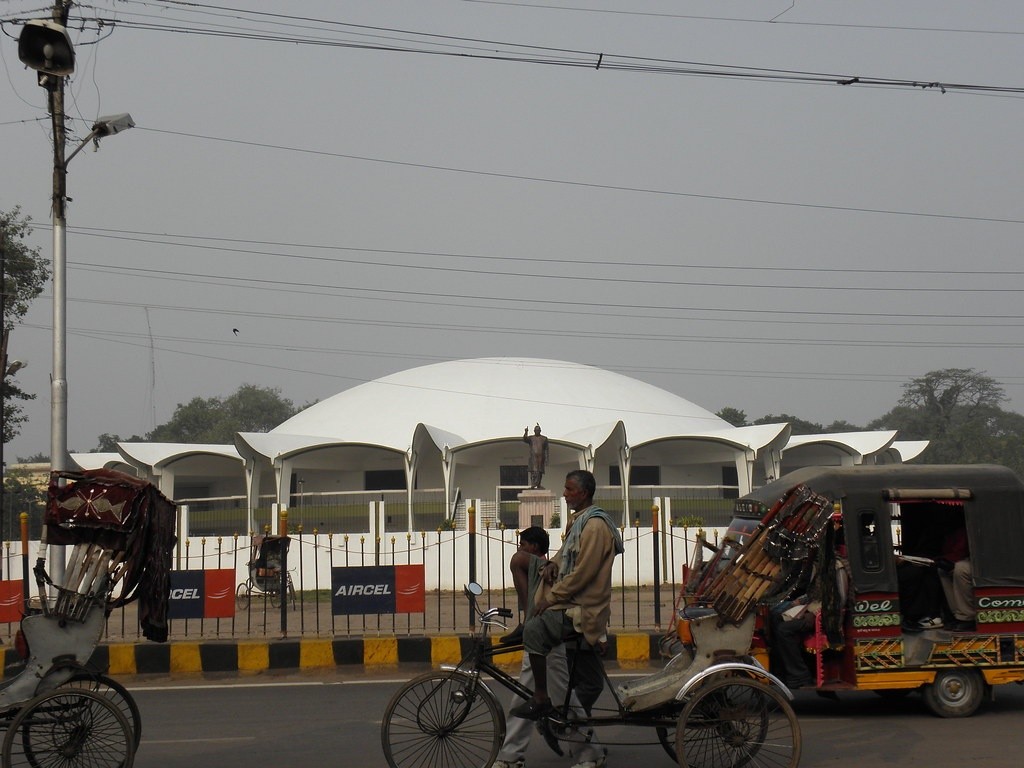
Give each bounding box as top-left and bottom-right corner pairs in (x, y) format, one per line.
(499, 469), (625, 719)
(484, 526), (607, 768)
(770, 536), (847, 686)
(523, 423), (549, 490)
(901, 525), (976, 632)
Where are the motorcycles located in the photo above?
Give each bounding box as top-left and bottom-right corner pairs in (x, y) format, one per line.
(658, 463), (1024, 720)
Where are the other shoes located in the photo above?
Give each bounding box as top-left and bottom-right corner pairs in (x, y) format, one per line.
(509, 697), (552, 719)
(571, 755), (606, 768)
(786, 675), (813, 688)
(944, 618), (976, 631)
(917, 617), (944, 628)
(499, 623), (525, 645)
(491, 760), (526, 768)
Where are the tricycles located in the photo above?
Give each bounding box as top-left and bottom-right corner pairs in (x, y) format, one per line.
(0, 466), (182, 768)
(380, 483), (844, 768)
(236, 536), (299, 612)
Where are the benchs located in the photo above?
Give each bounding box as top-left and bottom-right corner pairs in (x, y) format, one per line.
(14, 595), (106, 660)
(672, 595), (756, 656)
(894, 554), (934, 570)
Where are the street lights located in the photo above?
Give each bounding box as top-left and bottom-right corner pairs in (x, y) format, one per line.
(13, 17), (135, 610)
